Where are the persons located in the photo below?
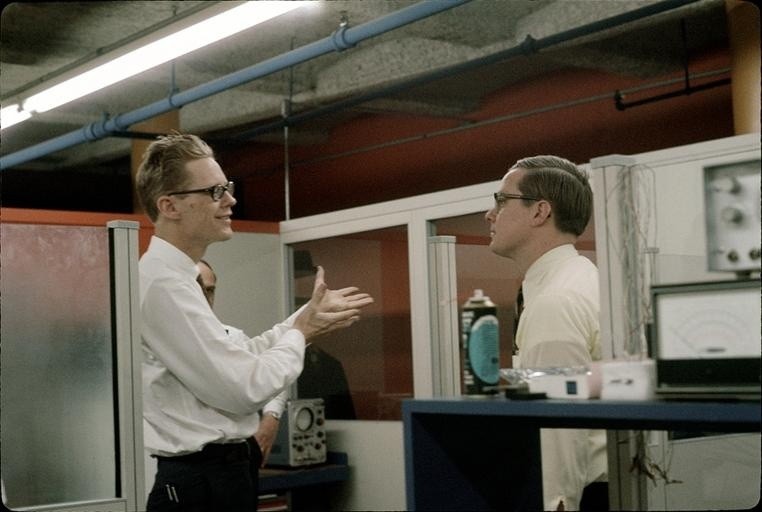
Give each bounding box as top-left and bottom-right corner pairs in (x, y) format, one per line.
(486, 154), (610, 512)
(193, 258), (289, 469)
(137, 131), (372, 511)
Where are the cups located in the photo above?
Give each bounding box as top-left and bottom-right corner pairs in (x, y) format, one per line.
(599, 358), (657, 405)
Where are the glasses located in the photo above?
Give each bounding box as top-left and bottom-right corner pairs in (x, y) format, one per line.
(494, 191), (542, 206)
(167, 180), (235, 201)
